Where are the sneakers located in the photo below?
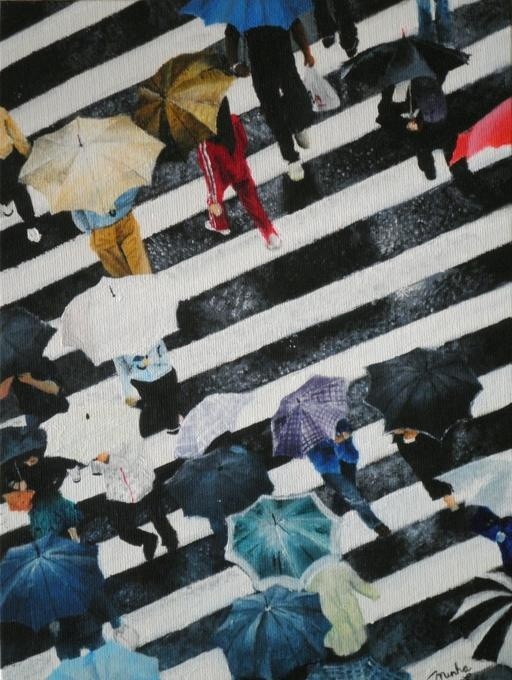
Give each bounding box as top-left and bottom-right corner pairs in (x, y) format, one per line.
(27, 228), (40, 242)
(287, 162), (305, 181)
(205, 220), (230, 236)
(264, 234), (282, 249)
(295, 130), (311, 149)
(3, 204), (14, 216)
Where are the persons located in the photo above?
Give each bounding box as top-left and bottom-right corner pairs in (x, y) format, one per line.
(0, 0), (512, 680)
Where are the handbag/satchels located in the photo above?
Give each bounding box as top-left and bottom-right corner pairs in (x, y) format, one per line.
(2, 482), (34, 511)
